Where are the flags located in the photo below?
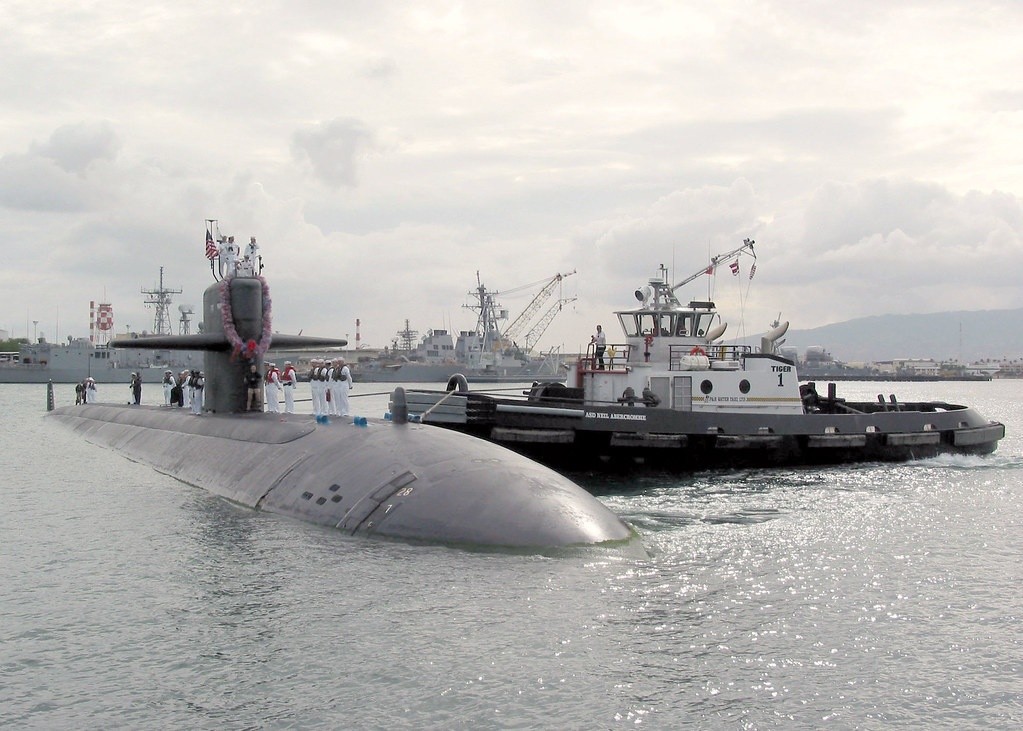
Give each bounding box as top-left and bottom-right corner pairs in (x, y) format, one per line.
(749, 263), (756, 280)
(206, 228), (218, 260)
(729, 259), (739, 276)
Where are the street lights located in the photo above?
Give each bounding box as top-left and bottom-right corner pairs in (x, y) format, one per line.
(33, 320), (38, 343)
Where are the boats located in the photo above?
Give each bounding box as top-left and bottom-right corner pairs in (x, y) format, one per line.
(911, 363), (1001, 380)
(388, 238), (1006, 463)
(351, 272), (570, 384)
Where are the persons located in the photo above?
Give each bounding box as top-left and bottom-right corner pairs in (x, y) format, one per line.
(642, 388), (662, 407)
(76, 377), (97, 406)
(217, 235), (259, 281)
(130, 372), (142, 404)
(591, 325), (606, 370)
(623, 387), (635, 407)
(804, 389), (817, 414)
(308, 357), (353, 418)
(281, 361), (296, 414)
(244, 365), (264, 412)
(162, 369), (205, 415)
(676, 317), (686, 337)
(264, 361), (283, 415)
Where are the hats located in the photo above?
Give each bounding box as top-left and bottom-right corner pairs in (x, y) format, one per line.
(284, 361), (291, 364)
(311, 356), (345, 363)
(264, 361), (276, 367)
(165, 370), (172, 374)
(189, 368), (200, 374)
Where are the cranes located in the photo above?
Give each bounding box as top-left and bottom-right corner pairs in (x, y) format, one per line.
(503, 269), (579, 354)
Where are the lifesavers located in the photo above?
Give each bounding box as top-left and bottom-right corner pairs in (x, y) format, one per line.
(445, 373), (468, 392)
(690, 347), (705, 356)
